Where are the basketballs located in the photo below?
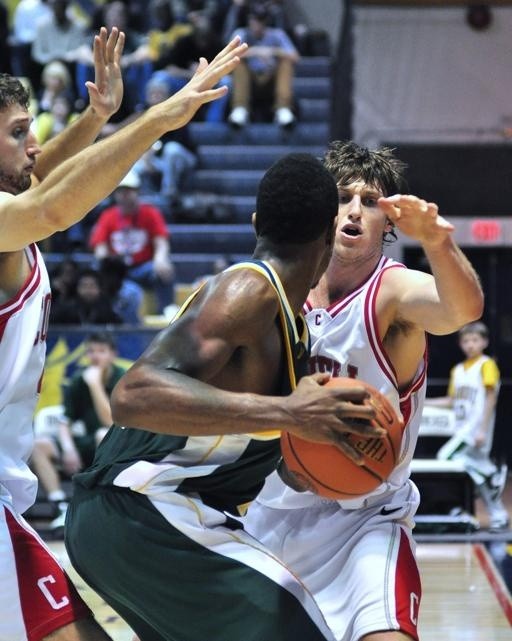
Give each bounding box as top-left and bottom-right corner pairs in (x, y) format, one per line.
(281, 378), (403, 502)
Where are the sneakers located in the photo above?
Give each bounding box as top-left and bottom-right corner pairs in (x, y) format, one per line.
(485, 463), (507, 501)
(489, 512), (509, 533)
(228, 106), (250, 128)
(275, 107), (296, 127)
(49, 497), (71, 533)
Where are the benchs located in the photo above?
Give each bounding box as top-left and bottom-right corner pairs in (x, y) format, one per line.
(409, 407), (473, 532)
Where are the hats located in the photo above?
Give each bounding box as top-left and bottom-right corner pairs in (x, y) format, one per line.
(118, 170), (142, 189)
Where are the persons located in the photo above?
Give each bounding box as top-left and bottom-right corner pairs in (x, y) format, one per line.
(1, 0), (512, 641)
